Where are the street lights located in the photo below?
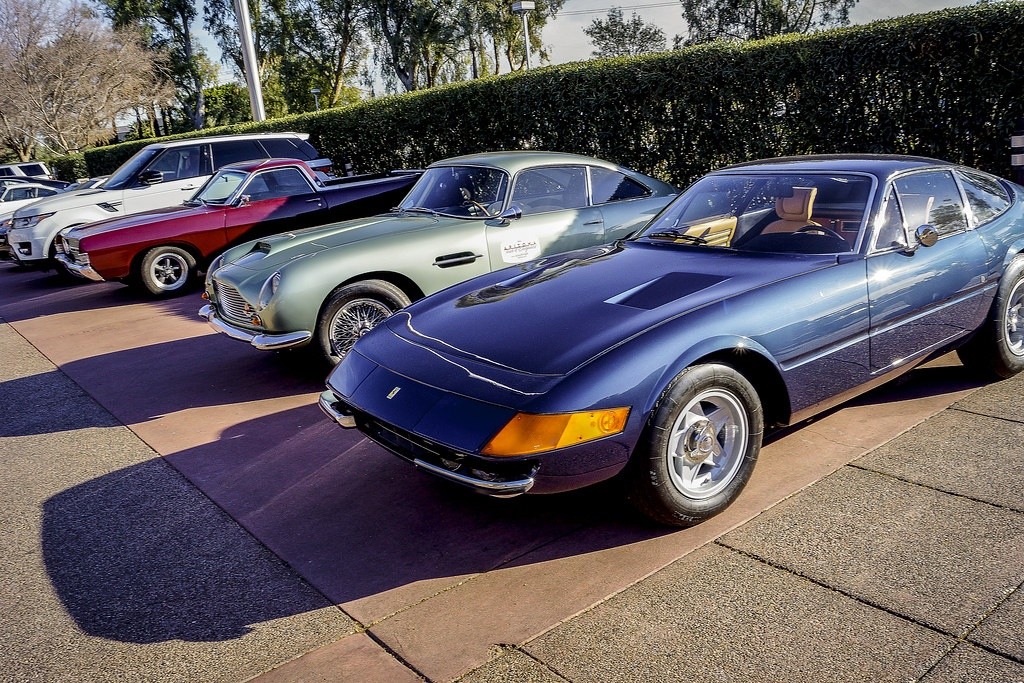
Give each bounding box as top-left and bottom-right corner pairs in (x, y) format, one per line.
(312, 89), (322, 113)
(511, 1), (536, 70)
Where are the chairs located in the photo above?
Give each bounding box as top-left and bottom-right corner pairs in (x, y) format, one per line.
(884, 195), (937, 245)
(759, 185), (824, 236)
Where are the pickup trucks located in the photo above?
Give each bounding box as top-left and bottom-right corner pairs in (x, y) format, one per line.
(53, 158), (428, 297)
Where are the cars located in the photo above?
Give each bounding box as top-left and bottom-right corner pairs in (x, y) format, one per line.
(316, 154), (1023, 525)
(197, 150), (681, 367)
(0, 176), (112, 254)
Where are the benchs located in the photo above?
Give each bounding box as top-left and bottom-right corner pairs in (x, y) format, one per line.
(560, 170), (630, 205)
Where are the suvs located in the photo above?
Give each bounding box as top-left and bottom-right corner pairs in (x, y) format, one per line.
(5, 131), (326, 281)
(0, 161), (55, 180)
